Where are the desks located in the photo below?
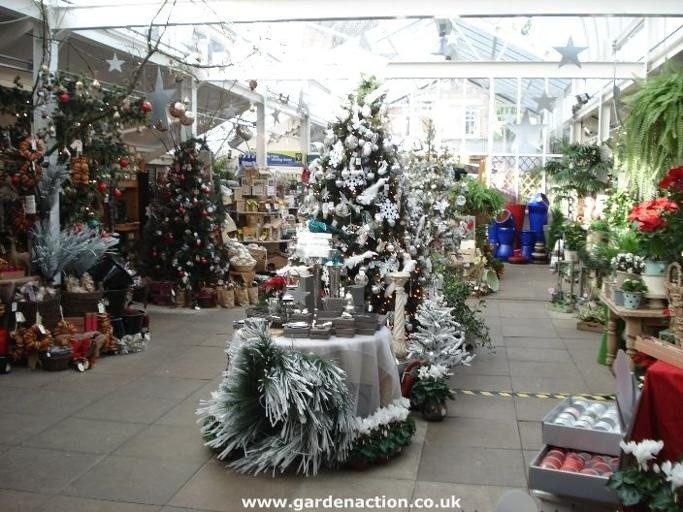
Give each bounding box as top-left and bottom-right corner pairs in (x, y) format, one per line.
(600, 295), (672, 378)
(230, 328), (395, 464)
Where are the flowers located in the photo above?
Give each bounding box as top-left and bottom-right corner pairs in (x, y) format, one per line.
(606, 437), (683, 512)
(414, 364), (455, 406)
(610, 253), (648, 292)
(631, 163), (683, 249)
(345, 398), (416, 464)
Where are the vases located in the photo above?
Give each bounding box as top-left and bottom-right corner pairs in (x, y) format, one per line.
(425, 397), (448, 422)
(623, 291), (642, 309)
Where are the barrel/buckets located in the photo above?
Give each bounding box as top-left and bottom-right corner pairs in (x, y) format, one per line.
(489, 201), (548, 262)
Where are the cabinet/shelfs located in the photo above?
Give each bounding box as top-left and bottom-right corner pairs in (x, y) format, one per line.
(237, 198), (296, 276)
